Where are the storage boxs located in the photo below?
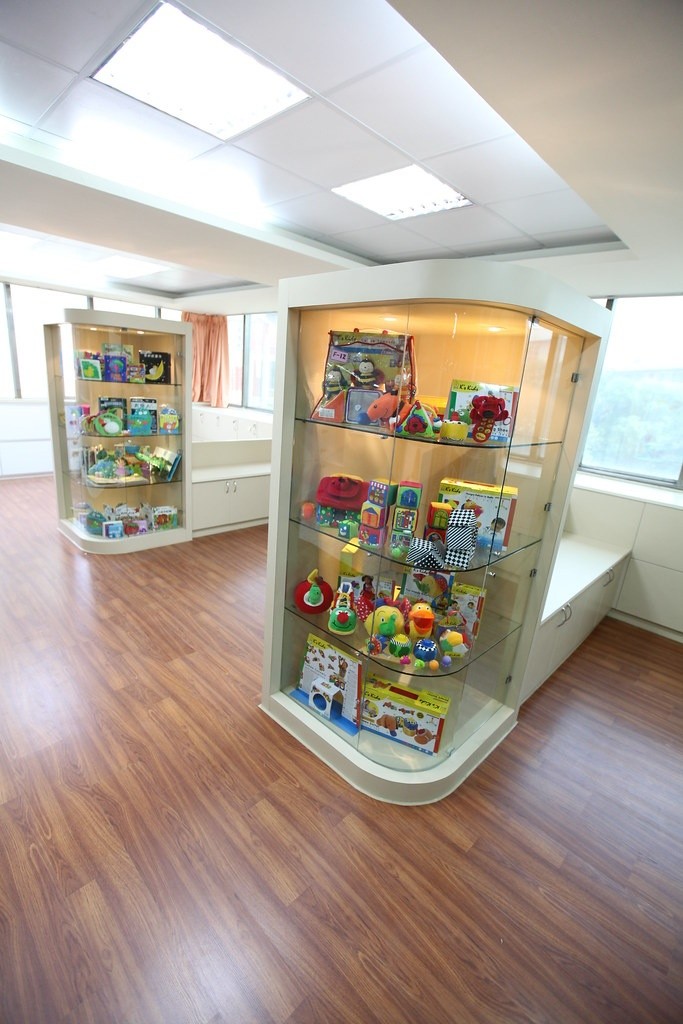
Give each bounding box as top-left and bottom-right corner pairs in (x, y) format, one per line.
(447, 582), (487, 640)
(340, 538), (363, 577)
(358, 671), (452, 758)
(400, 561), (459, 618)
(438, 477), (519, 551)
(443, 378), (520, 444)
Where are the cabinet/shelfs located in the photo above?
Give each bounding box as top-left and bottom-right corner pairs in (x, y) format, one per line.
(192, 404), (273, 441)
(37, 308), (193, 556)
(520, 556), (628, 703)
(191, 475), (270, 531)
(259, 259), (614, 806)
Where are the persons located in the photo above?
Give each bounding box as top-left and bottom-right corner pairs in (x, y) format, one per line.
(446, 602), (479, 622)
(485, 518), (507, 538)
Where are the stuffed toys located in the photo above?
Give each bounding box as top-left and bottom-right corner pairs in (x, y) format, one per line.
(293, 568), (440, 672)
(311, 329), (440, 439)
